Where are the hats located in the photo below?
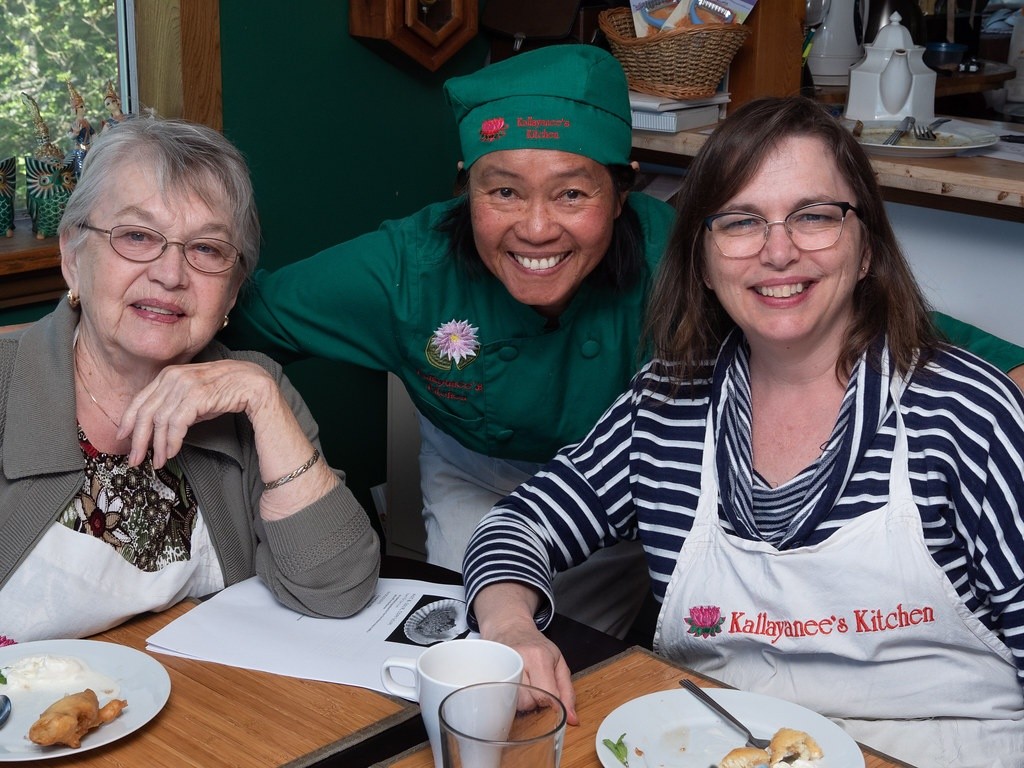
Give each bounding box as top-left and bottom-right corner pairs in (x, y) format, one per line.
(443, 43), (633, 171)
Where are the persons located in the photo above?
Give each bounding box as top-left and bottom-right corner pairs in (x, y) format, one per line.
(461, 98), (1024, 768)
(0, 118), (382, 648)
(212, 44), (1024, 645)
(64, 79), (95, 183)
(102, 80), (135, 134)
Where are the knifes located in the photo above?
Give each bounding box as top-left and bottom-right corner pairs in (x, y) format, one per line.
(884, 116), (916, 145)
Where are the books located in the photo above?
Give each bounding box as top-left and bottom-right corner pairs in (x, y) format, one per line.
(628, 91), (732, 132)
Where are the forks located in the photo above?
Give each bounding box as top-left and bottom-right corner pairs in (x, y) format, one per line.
(912, 118), (953, 140)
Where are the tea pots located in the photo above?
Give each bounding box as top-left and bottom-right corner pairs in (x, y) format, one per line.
(841, 10), (937, 121)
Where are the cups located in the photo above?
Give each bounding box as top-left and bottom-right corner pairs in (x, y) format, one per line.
(437, 680), (567, 768)
(381, 638), (522, 768)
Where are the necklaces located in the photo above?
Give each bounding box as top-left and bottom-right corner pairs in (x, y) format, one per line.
(75, 339), (156, 480)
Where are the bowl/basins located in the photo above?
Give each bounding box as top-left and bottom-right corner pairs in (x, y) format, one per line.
(922, 41), (969, 73)
(403, 599), (469, 645)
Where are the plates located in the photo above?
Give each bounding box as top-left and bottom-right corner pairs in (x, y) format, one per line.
(594, 687), (866, 768)
(836, 118), (1001, 158)
(0, 639), (172, 762)
(995, 103), (1024, 117)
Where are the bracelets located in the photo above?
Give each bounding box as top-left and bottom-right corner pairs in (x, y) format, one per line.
(262, 449), (320, 490)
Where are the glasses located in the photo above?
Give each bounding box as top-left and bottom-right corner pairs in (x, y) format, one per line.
(705, 201), (860, 258)
(77, 221), (244, 274)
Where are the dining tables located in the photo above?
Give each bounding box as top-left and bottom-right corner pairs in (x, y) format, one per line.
(0, 555), (916, 768)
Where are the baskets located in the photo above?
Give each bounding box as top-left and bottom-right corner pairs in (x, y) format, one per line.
(598, 7), (753, 100)
